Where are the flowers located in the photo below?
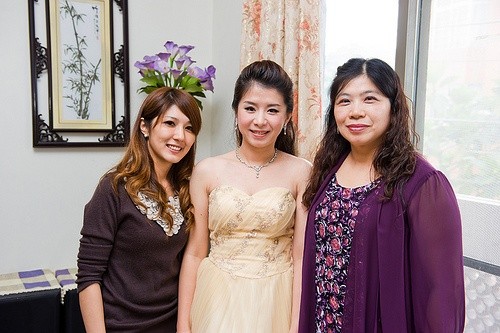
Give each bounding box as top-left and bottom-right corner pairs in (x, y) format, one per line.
(133, 41), (216, 110)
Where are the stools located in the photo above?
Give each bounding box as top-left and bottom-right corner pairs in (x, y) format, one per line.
(55, 268), (86, 333)
(0, 270), (61, 333)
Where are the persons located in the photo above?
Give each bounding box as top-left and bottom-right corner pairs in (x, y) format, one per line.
(75, 87), (201, 333)
(298, 58), (465, 333)
(177, 60), (313, 333)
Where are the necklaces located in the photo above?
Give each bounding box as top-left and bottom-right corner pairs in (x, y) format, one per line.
(236, 145), (278, 178)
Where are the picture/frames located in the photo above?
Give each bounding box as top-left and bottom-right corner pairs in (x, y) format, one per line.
(28, 0), (131, 146)
